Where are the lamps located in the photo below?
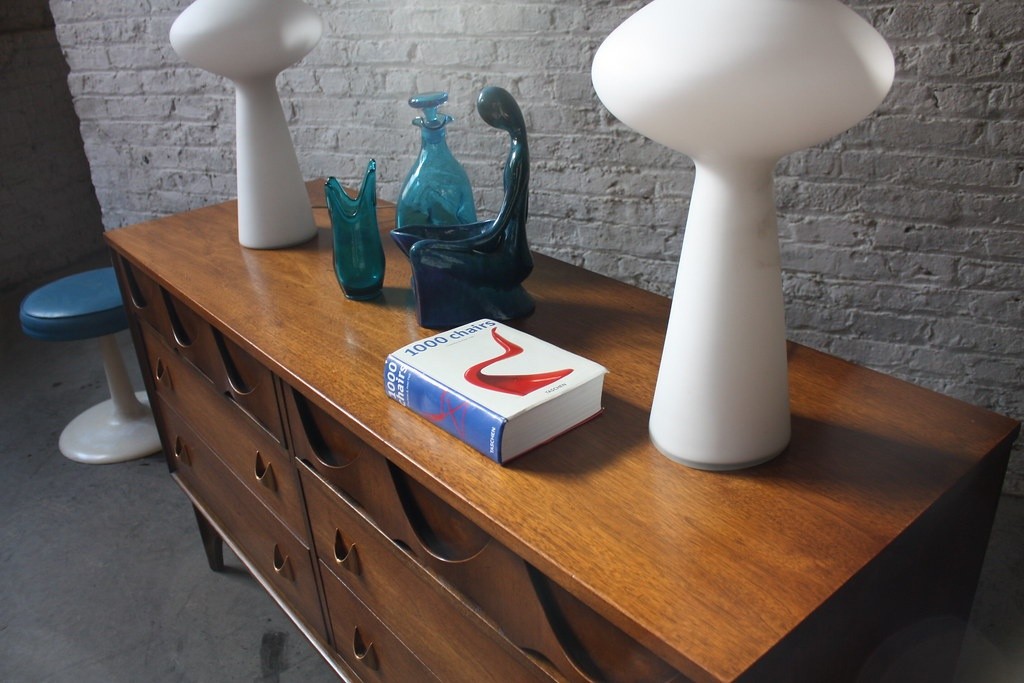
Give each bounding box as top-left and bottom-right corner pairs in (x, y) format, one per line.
(590, 1), (897, 473)
(166, 0), (329, 250)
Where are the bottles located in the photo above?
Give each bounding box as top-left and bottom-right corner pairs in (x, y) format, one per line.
(395, 94), (478, 231)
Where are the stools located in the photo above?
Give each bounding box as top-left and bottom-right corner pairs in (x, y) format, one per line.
(17, 265), (168, 466)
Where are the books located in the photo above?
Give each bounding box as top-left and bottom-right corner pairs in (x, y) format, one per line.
(382, 319), (610, 467)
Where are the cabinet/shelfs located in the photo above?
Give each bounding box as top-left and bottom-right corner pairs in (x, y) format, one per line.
(97, 167), (1023, 683)
